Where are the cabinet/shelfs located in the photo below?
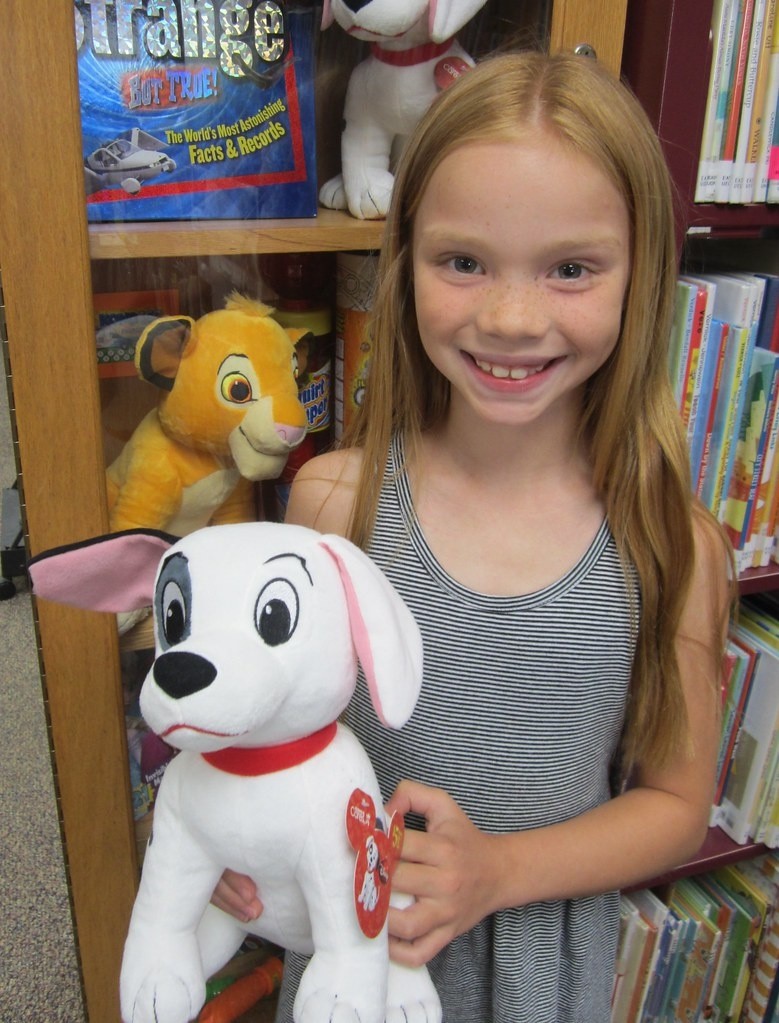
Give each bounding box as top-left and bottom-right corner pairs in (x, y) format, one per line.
(0, 1), (779, 1023)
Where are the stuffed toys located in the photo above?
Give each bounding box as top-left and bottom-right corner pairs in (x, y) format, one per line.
(320, 0), (490, 221)
(28, 522), (442, 1023)
(105, 291), (309, 634)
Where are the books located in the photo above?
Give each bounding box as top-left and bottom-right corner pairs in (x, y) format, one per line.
(210, 53), (741, 1023)
(694, 0), (779, 203)
(611, 602), (779, 1023)
(663, 274), (779, 579)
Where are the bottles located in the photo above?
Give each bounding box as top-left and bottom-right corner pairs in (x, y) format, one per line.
(258, 254), (336, 480)
